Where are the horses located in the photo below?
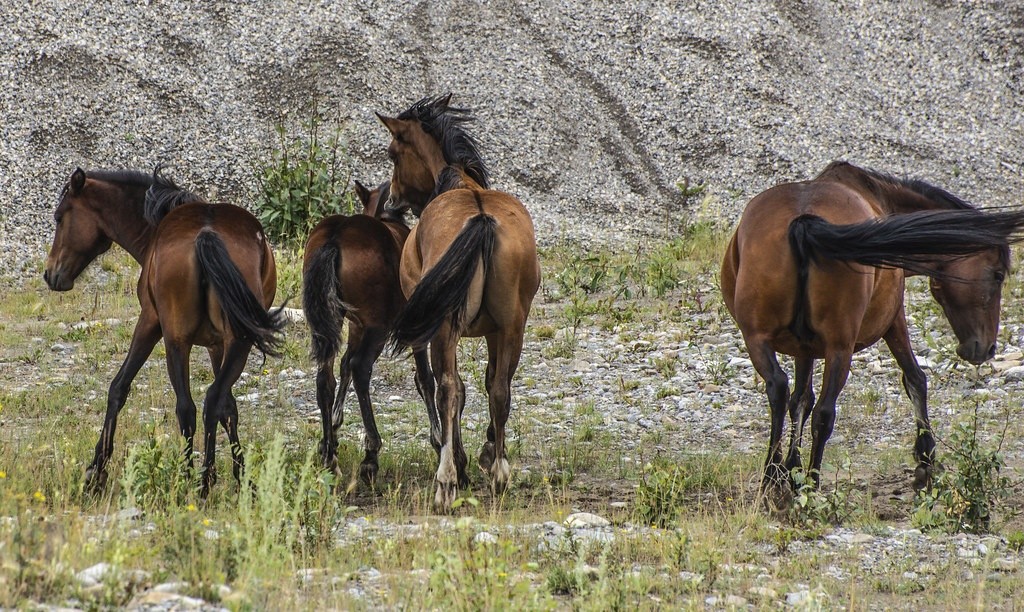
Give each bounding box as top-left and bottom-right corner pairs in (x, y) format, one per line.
(374, 92), (542, 517)
(303, 179), (410, 490)
(718, 159), (1024, 502)
(42, 163), (295, 501)
